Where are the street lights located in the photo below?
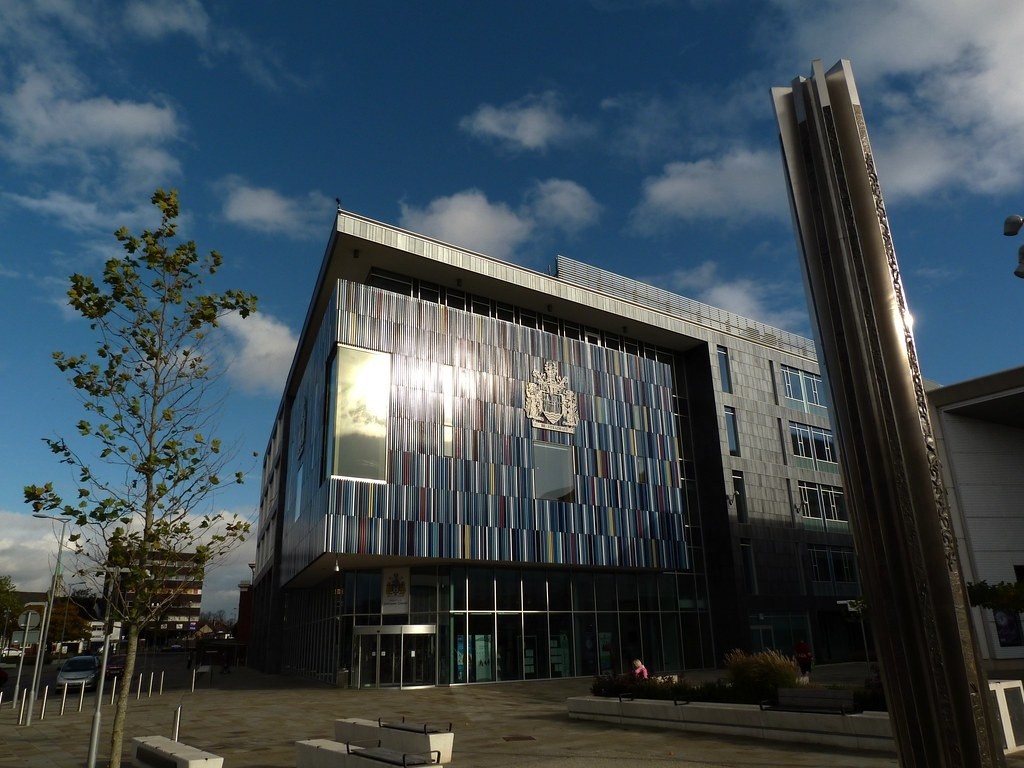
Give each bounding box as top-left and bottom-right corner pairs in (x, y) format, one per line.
(57, 581), (88, 667)
(33, 514), (72, 701)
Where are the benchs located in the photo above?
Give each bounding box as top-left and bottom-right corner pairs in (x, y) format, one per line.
(334, 716), (455, 764)
(131, 735), (224, 768)
(294, 738), (443, 768)
(759, 689), (854, 715)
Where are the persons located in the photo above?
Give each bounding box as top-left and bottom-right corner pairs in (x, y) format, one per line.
(791, 637), (814, 677)
(631, 658), (649, 686)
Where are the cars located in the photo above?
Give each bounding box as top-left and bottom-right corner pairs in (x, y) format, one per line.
(55, 655), (102, 694)
(106, 653), (129, 681)
(0, 647), (26, 658)
